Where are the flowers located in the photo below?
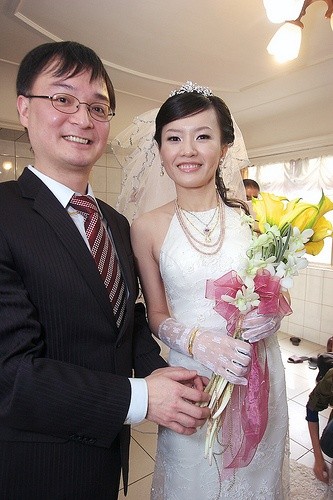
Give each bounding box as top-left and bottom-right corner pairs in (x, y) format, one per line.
(195, 188), (333, 466)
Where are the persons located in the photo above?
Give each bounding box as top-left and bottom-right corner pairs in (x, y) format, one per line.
(0, 41), (210, 500)
(128, 92), (287, 500)
(240, 178), (260, 201)
(305, 367), (333, 500)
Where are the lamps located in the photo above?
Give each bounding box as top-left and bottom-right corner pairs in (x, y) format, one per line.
(262, 0), (333, 61)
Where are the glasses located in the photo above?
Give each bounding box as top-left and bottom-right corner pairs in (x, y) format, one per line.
(24, 92), (117, 122)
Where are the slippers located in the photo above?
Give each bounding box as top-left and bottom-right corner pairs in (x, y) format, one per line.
(287, 355), (318, 370)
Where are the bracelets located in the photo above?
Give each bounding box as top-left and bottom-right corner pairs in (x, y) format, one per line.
(188, 327), (202, 359)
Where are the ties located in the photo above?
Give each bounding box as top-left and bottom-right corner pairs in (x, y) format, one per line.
(69, 195), (128, 330)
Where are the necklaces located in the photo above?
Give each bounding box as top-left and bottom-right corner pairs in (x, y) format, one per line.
(173, 190), (226, 255)
